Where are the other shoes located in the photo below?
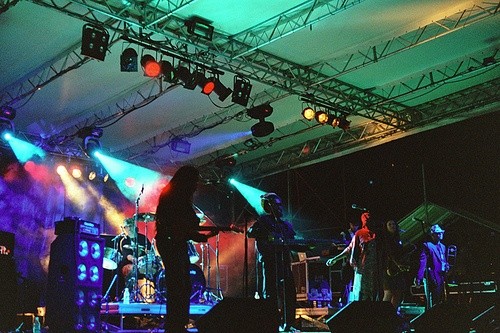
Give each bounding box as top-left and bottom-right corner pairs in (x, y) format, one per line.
(279, 323), (296, 332)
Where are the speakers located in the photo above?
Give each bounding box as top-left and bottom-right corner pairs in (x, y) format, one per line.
(195, 296), (280, 333)
(470, 304), (500, 333)
(410, 302), (470, 333)
(325, 300), (403, 333)
(45, 232), (106, 333)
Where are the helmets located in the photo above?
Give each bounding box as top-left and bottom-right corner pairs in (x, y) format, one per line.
(261, 193), (281, 213)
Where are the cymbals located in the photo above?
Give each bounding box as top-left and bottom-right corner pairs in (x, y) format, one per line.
(132, 212), (155, 222)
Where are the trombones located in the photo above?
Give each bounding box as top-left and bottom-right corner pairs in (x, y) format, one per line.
(443, 244), (458, 298)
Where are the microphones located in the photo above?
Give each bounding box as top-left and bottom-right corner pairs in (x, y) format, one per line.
(352, 204), (366, 211)
(412, 217), (425, 224)
(261, 195), (271, 201)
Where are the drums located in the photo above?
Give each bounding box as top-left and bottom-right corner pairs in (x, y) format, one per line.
(138, 255), (159, 276)
(102, 246), (122, 271)
(186, 241), (200, 264)
(157, 263), (207, 303)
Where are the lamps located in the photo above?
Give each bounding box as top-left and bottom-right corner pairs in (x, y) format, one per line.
(120, 43), (253, 107)
(53, 161), (109, 184)
(301, 97), (351, 132)
(79, 22), (110, 62)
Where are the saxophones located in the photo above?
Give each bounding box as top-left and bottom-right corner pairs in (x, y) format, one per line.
(385, 241), (417, 277)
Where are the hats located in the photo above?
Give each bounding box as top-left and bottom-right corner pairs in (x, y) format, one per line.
(430, 224), (445, 234)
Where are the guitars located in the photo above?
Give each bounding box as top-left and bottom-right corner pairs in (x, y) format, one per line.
(166, 215), (244, 245)
(255, 232), (315, 258)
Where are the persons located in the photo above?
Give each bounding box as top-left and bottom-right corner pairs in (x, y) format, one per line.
(153, 165), (219, 333)
(417, 224), (449, 306)
(248, 194), (296, 326)
(119, 220), (151, 289)
(326, 212), (408, 305)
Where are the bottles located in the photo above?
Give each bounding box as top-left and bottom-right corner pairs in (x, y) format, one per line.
(254, 292), (260, 299)
(33, 317), (40, 333)
(123, 287), (130, 305)
